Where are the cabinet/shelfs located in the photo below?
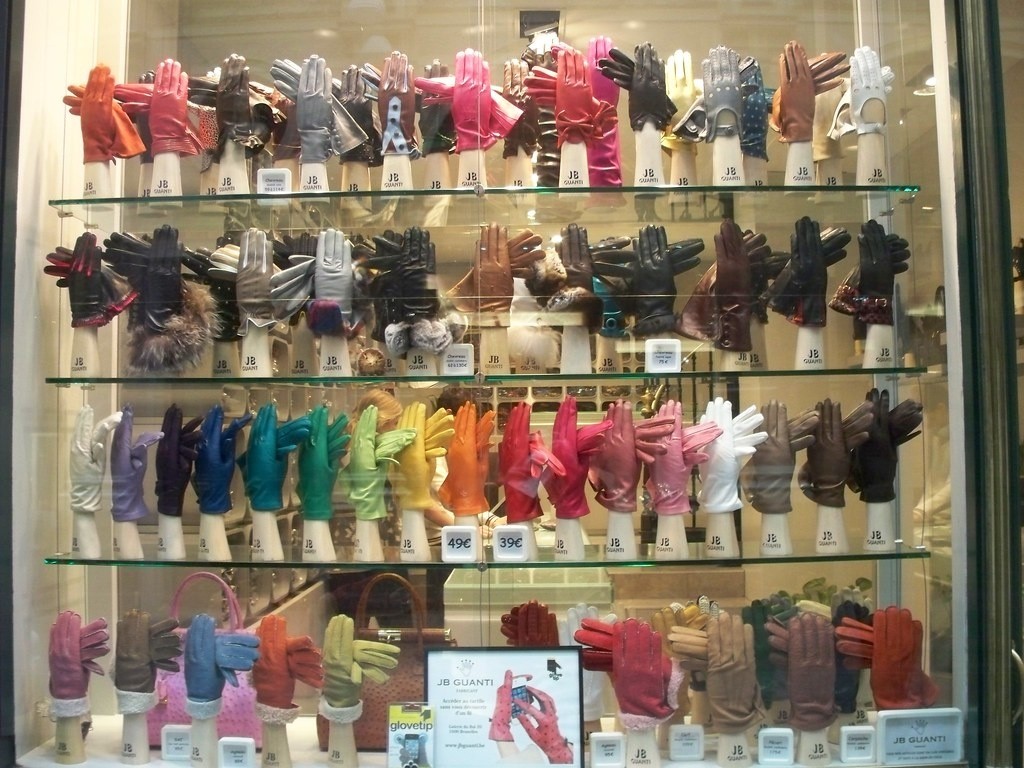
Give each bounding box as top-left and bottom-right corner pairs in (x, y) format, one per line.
(10, 187), (979, 768)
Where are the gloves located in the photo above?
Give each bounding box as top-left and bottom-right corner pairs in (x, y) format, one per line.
(254, 617), (324, 725)
(113, 610), (181, 713)
(183, 614), (261, 720)
(45, 216), (909, 365)
(320, 614), (399, 722)
(65, 35), (896, 219)
(47, 611), (108, 716)
(502, 587), (939, 733)
(70, 389), (923, 521)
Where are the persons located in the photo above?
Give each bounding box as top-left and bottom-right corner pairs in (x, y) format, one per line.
(425, 386), (474, 629)
(323, 388), (414, 630)
(916, 387), (952, 686)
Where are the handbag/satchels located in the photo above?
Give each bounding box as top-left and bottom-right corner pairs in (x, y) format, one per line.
(145, 573), (264, 749)
(317, 573), (457, 748)
